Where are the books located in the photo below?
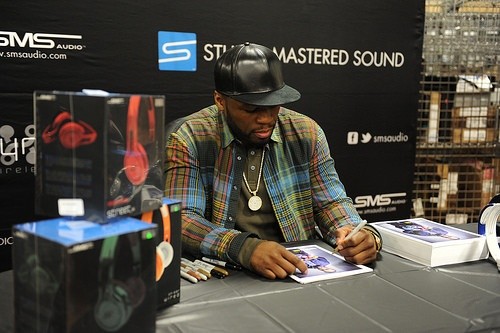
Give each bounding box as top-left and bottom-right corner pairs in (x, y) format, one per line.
(368, 218), (490, 268)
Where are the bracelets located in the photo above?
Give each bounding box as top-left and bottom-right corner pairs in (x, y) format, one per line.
(370, 230), (382, 252)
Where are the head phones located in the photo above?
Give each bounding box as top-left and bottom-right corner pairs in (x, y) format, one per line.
(17, 95), (174, 332)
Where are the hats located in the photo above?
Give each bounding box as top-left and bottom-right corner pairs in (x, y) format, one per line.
(214, 42), (301, 106)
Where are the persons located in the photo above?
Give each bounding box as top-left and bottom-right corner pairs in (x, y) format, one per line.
(387, 221), (460, 240)
(287, 249), (336, 274)
(161, 42), (382, 278)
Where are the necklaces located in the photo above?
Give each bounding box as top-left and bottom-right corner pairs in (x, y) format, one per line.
(242, 147), (266, 211)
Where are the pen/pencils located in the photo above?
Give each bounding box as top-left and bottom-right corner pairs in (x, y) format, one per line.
(332, 220), (367, 253)
(180, 257), (241, 284)
